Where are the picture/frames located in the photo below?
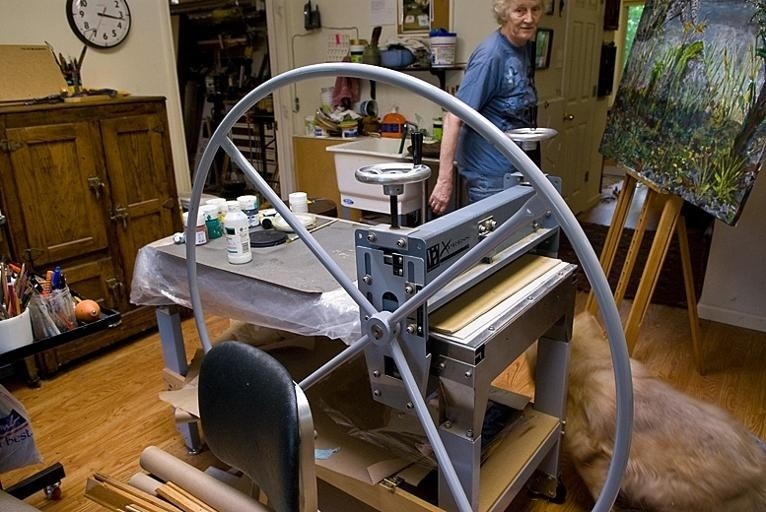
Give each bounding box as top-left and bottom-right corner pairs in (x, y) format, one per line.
(533, 28), (554, 71)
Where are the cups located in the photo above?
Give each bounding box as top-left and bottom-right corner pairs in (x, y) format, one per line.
(0, 286), (80, 355)
(320, 85), (335, 106)
(349, 37), (365, 65)
(355, 99), (379, 118)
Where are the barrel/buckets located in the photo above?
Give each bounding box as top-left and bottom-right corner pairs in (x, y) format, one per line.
(429, 28), (457, 68)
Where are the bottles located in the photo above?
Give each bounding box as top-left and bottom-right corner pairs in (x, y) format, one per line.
(432, 116), (444, 141)
(181, 191), (308, 265)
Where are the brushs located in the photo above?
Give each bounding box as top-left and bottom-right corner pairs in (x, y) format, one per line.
(370, 26), (382, 48)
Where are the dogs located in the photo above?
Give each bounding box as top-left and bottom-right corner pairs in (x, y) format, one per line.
(524, 309), (766, 512)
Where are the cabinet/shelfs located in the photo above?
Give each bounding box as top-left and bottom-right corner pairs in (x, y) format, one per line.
(180, 1), (274, 109)
(395, 0), (450, 37)
(0, 92), (195, 380)
(289, 132), (364, 223)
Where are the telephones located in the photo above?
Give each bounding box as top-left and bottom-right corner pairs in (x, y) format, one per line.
(303, 0), (320, 31)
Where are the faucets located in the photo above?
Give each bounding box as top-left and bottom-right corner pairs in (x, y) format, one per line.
(403, 121), (418, 134)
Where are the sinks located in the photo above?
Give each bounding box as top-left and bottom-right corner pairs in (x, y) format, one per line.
(325, 137), (423, 216)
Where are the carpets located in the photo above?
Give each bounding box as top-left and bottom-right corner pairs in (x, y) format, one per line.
(561, 220), (713, 312)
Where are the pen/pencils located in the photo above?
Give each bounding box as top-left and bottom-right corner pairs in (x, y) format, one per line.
(44, 40), (88, 72)
(0, 261), (75, 340)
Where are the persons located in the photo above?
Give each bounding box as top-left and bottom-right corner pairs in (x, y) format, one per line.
(428, 0), (544, 216)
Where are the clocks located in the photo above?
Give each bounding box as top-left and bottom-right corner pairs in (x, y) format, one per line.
(63, 0), (133, 51)
